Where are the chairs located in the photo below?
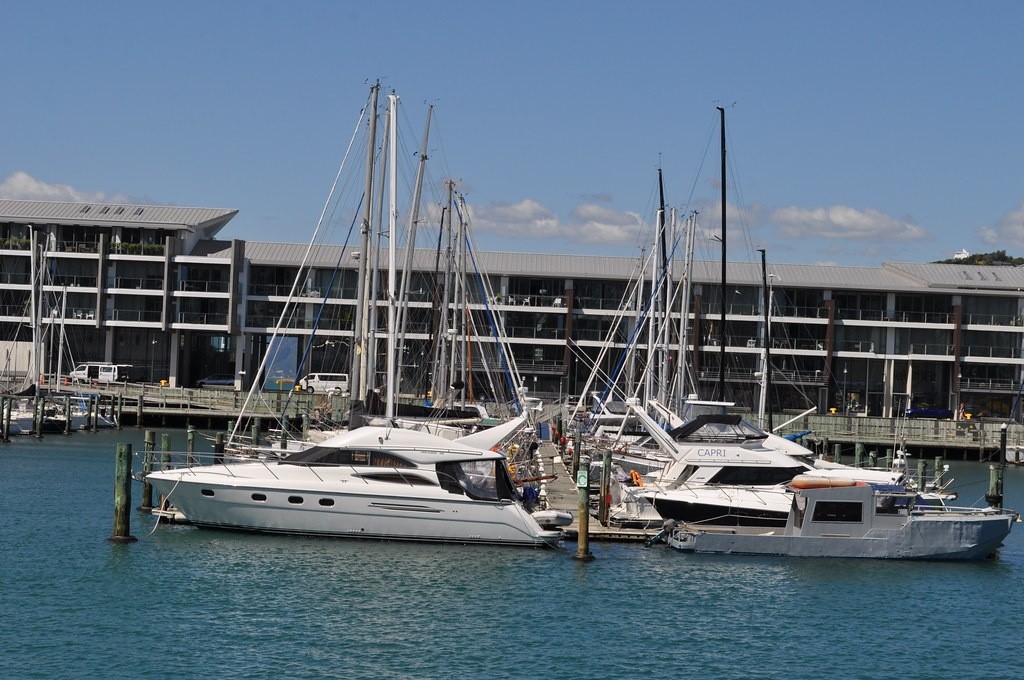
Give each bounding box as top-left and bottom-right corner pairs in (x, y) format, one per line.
(508, 296), (516, 305)
(74, 310), (83, 318)
(523, 296), (531, 306)
(64, 241), (72, 252)
(86, 310), (95, 320)
(747, 339), (756, 348)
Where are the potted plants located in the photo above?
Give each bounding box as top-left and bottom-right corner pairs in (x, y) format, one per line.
(0, 235), (29, 250)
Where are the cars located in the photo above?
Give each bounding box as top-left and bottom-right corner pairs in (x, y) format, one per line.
(193, 371), (235, 388)
(903, 402), (954, 419)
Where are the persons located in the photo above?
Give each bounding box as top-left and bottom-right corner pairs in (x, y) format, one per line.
(959, 403), (966, 421)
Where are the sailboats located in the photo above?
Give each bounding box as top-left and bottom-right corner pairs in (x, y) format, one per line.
(130, 77), (1023, 569)
(0, 221), (119, 438)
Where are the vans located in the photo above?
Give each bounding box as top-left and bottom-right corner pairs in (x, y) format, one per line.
(67, 362), (119, 386)
(298, 372), (351, 396)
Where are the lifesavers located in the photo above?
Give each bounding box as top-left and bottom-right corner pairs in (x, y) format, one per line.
(630, 469), (643, 487)
